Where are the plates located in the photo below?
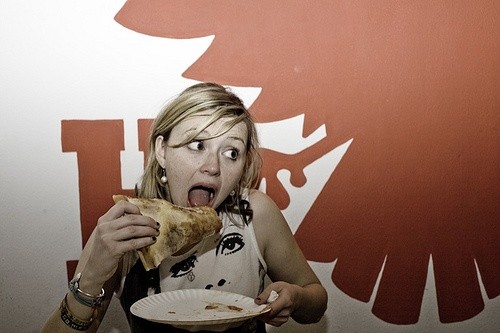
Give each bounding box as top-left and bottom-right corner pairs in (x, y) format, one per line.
(129, 287), (273, 327)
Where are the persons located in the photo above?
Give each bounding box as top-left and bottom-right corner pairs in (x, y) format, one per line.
(37, 82), (328, 333)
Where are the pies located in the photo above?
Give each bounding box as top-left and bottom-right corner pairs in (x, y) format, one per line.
(111, 195), (223, 271)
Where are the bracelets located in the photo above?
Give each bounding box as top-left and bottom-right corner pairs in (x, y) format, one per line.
(68, 272), (105, 308)
(59, 293), (94, 331)
